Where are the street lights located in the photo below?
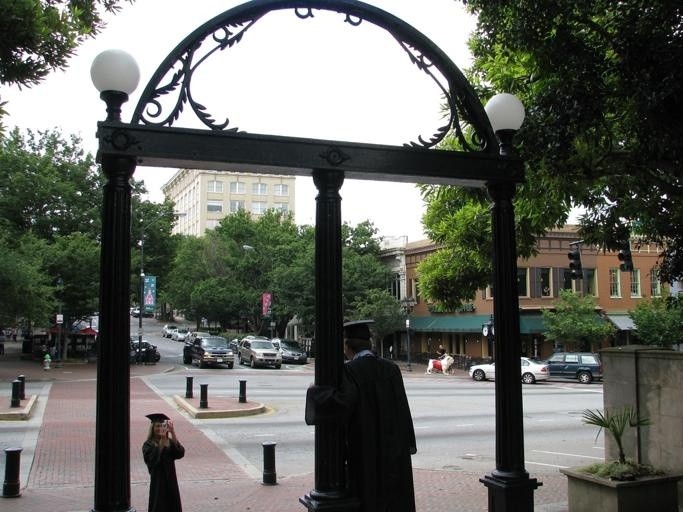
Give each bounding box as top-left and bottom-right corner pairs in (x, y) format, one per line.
(243, 244), (276, 339)
(139, 210), (187, 341)
(402, 297), (416, 372)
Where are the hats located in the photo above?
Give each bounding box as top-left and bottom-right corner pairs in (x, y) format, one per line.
(344, 318), (376, 341)
(144, 413), (171, 424)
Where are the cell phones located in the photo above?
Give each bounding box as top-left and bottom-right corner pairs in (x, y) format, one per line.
(162, 421), (168, 432)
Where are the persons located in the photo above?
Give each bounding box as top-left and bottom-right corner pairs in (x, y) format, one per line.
(6, 326), (17, 342)
(145, 287), (153, 304)
(305, 319), (418, 511)
(461, 352), (472, 372)
(437, 344), (446, 359)
(142, 413), (184, 512)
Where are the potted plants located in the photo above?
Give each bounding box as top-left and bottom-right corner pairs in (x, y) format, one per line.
(558, 407), (682, 512)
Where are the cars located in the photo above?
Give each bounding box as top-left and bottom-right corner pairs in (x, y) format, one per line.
(130, 307), (154, 318)
(162, 324), (307, 369)
(466, 356), (552, 385)
(130, 339), (159, 362)
(130, 333), (140, 344)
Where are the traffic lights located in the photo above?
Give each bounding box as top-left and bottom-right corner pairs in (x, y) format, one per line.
(616, 240), (633, 272)
(567, 249), (580, 279)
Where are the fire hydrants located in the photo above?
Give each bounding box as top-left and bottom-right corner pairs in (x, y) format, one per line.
(43, 354), (52, 370)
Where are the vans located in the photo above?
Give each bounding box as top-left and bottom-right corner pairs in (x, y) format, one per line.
(543, 353), (602, 383)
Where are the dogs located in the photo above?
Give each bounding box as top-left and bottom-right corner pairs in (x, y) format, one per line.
(427, 356), (455, 376)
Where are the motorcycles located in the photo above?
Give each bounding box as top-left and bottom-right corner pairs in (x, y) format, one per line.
(134, 345), (159, 364)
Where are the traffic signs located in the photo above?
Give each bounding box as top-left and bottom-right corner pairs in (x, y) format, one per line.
(632, 220), (644, 230)
